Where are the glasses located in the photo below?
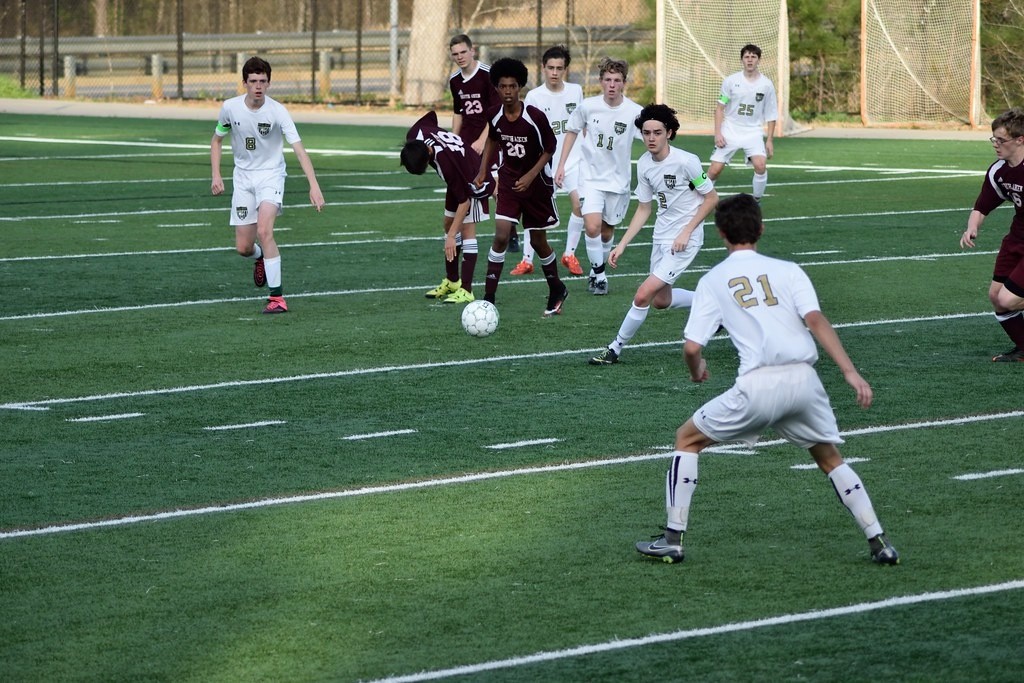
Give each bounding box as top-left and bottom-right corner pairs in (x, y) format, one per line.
(990, 136), (1017, 144)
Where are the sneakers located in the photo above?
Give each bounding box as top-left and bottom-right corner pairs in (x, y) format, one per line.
(254, 244), (267, 287)
(636, 526), (685, 563)
(715, 324), (724, 333)
(506, 235), (520, 252)
(263, 295), (287, 313)
(544, 286), (569, 317)
(871, 546), (899, 567)
(510, 259), (533, 276)
(594, 279), (609, 295)
(426, 278), (462, 299)
(588, 276), (596, 292)
(443, 287), (475, 304)
(589, 346), (619, 365)
(993, 347), (1024, 362)
(561, 252), (583, 275)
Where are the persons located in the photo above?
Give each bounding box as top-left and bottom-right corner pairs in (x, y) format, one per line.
(210, 56), (324, 314)
(706, 44), (777, 204)
(401, 111), (495, 303)
(587, 103), (722, 365)
(510, 47), (586, 276)
(447, 34), (520, 253)
(553, 57), (646, 295)
(472, 57), (568, 318)
(958, 108), (1024, 362)
(635, 192), (899, 566)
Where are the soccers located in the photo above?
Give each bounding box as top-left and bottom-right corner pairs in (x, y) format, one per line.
(461, 300), (500, 337)
